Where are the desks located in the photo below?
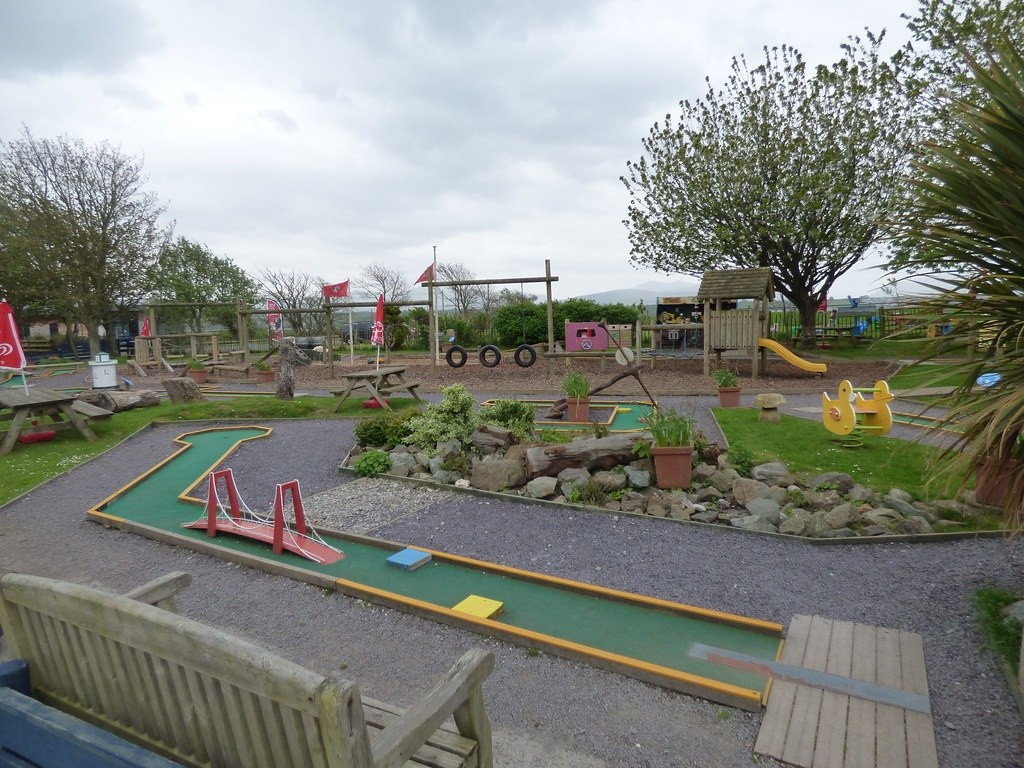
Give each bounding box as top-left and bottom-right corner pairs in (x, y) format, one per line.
(791, 327), (856, 349)
(0, 386), (98, 457)
(332, 366), (421, 414)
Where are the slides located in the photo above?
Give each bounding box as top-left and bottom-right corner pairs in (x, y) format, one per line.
(758, 339), (827, 373)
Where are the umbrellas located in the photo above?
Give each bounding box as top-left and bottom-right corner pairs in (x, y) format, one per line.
(816, 290), (827, 338)
(0, 298), (30, 397)
(371, 294), (384, 390)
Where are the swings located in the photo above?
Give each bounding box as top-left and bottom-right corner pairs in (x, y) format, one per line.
(514, 283), (537, 368)
(446, 285), (467, 368)
(479, 284), (501, 367)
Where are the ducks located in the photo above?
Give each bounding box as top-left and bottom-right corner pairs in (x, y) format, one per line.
(822, 379), (857, 436)
(856, 380), (895, 435)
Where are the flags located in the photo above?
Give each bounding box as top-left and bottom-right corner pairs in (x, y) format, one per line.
(140, 315), (150, 337)
(323, 280), (350, 297)
(413, 263), (436, 285)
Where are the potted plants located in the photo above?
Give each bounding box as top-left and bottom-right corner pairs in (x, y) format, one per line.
(440, 334), (453, 353)
(250, 360), (275, 383)
(634, 394), (700, 489)
(561, 371), (591, 422)
(182, 355), (209, 384)
(711, 368), (742, 407)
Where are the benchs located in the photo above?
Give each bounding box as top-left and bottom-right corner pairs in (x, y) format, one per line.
(329, 381), (377, 398)
(377, 382), (419, 398)
(791, 337), (836, 342)
(24, 362), (84, 374)
(816, 334), (866, 344)
(58, 341), (105, 360)
(0, 567), (495, 768)
(70, 400), (115, 425)
